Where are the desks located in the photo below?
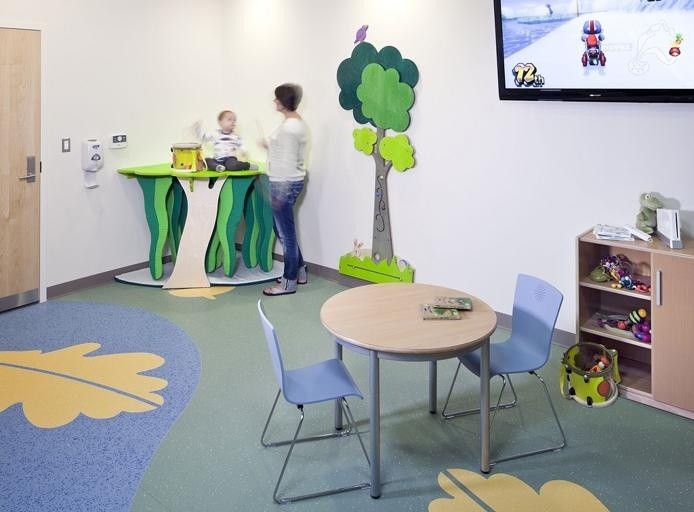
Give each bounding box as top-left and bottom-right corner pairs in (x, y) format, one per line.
(317, 282), (499, 499)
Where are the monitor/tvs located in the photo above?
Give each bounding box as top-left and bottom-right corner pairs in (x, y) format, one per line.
(494, 0), (694, 102)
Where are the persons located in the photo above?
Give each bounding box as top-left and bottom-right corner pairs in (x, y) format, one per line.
(254, 81), (309, 297)
(191, 108), (261, 174)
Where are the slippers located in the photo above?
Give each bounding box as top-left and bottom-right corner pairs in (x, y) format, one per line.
(277, 278), (307, 284)
(263, 288), (296, 296)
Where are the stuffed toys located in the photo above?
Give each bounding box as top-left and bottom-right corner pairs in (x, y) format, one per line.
(636, 192), (663, 234)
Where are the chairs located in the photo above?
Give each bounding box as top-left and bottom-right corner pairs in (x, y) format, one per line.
(440, 272), (566, 467)
(254, 297), (372, 504)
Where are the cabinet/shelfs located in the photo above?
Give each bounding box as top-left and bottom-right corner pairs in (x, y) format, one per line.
(575, 226), (650, 407)
(650, 250), (693, 420)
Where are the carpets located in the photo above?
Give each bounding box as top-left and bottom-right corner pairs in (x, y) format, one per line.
(0, 302), (205, 511)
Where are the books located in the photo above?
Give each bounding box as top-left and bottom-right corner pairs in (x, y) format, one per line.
(422, 303), (461, 320)
(593, 223), (632, 239)
(432, 296), (472, 310)
(595, 234), (635, 242)
